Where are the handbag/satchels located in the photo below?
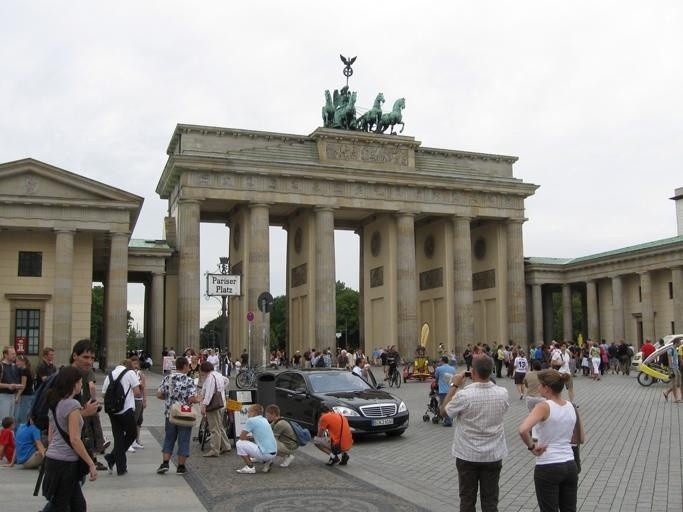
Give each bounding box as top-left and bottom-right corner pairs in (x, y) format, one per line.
(75, 446), (96, 481)
(169, 401), (196, 427)
(205, 391), (224, 412)
(571, 445), (581, 475)
(330, 437), (341, 455)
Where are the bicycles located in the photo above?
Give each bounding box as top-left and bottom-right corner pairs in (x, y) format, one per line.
(233, 362), (264, 390)
(193, 394), (230, 455)
(384, 361), (401, 388)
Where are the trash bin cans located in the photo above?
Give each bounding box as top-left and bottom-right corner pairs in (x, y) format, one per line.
(257, 372), (278, 417)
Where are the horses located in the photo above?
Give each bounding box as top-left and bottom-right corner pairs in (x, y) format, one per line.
(333, 92), (358, 131)
(380, 98), (406, 135)
(322, 89), (335, 128)
(356, 93), (385, 133)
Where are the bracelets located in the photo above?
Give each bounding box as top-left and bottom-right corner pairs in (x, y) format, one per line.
(526, 444), (534, 450)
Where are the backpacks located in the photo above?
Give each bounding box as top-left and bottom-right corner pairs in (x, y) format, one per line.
(274, 417), (312, 447)
(105, 370), (130, 413)
(30, 365), (72, 431)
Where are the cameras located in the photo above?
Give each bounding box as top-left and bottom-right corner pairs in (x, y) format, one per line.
(90, 399), (102, 412)
(465, 370), (471, 377)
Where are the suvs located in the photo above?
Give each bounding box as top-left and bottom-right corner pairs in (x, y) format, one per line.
(631, 332), (682, 372)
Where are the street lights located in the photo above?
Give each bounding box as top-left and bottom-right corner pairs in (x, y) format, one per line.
(336, 329), (341, 347)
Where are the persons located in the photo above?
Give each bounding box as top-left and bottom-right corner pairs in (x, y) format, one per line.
(516, 369), (585, 512)
(437, 353), (509, 512)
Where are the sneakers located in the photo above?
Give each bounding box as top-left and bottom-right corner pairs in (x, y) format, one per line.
(340, 453), (350, 465)
(104, 440), (143, 474)
(203, 444), (259, 463)
(176, 466), (186, 475)
(280, 454), (294, 467)
(236, 466), (256, 474)
(156, 461), (168, 474)
(326, 456), (340, 466)
(261, 459), (272, 473)
(383, 367), (682, 403)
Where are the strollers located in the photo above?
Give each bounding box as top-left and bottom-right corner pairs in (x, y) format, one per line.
(422, 379), (443, 426)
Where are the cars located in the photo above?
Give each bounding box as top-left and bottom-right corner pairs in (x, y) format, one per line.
(249, 368), (410, 439)
